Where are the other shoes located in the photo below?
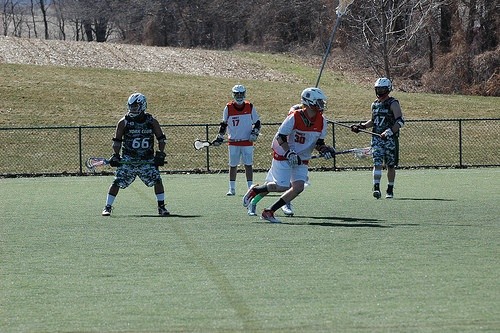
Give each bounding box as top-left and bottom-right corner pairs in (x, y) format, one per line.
(226, 188), (235, 195)
(281, 205), (294, 215)
(247, 203), (257, 216)
(372, 183), (381, 199)
(386, 183), (393, 198)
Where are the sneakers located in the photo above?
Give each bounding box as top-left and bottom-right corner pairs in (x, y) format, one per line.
(157, 200), (170, 215)
(261, 208), (281, 223)
(242, 183), (259, 207)
(101, 204), (114, 216)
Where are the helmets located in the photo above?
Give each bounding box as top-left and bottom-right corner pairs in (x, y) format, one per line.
(232, 84), (246, 105)
(374, 78), (392, 92)
(127, 93), (147, 118)
(288, 104), (303, 115)
(301, 87), (328, 107)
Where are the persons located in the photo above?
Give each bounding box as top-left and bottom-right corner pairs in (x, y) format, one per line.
(241, 87), (337, 223)
(349, 78), (405, 198)
(211, 85), (262, 196)
(102, 92), (170, 216)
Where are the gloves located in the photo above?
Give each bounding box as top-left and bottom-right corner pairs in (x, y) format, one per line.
(283, 149), (301, 165)
(249, 128), (259, 142)
(319, 146), (336, 160)
(351, 121), (366, 133)
(380, 127), (394, 140)
(154, 151), (166, 166)
(214, 134), (224, 146)
(109, 153), (123, 167)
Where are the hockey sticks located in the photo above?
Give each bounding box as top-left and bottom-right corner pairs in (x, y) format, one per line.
(85, 157), (168, 168)
(315, 0), (355, 88)
(194, 139), (248, 150)
(288, 146), (380, 166)
(325, 117), (390, 140)
